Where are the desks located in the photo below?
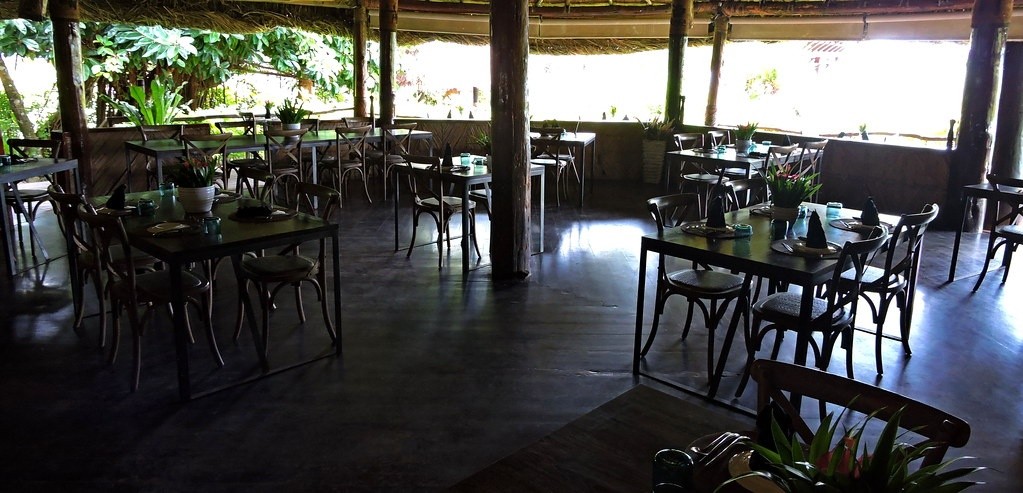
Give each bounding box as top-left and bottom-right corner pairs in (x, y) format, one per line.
(667, 141), (824, 207)
(446, 383), (812, 493)
(393, 155), (545, 272)
(634, 201), (927, 415)
(948, 183), (1023, 282)
(0, 157), (88, 275)
(530, 132), (597, 209)
(124, 128), (434, 210)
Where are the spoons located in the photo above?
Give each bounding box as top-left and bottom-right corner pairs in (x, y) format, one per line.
(690, 431), (731, 456)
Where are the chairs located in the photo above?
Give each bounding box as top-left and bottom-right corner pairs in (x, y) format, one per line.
(0, 113), (1023, 493)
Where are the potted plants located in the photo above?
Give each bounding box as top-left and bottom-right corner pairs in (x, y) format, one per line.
(274, 99), (313, 139)
(635, 108), (676, 185)
(750, 161), (823, 229)
(264, 100), (275, 118)
(732, 121), (760, 152)
(467, 130), (492, 173)
(162, 156), (223, 213)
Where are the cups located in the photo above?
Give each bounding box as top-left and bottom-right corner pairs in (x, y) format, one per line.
(651, 448), (694, 493)
(799, 204), (808, 219)
(0, 155), (11, 174)
(159, 182), (175, 197)
(827, 201), (843, 216)
(717, 145), (726, 158)
(137, 198), (157, 217)
(200, 216), (223, 243)
(473, 158), (483, 174)
(460, 152), (471, 167)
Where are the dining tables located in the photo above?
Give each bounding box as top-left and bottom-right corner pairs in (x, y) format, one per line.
(65, 186), (343, 406)
